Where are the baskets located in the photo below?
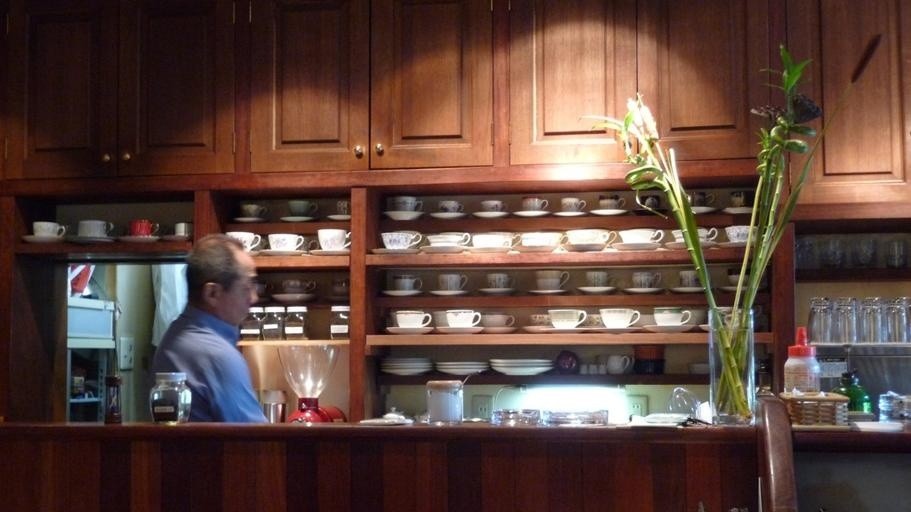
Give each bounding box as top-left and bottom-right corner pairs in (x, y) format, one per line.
(780, 390), (854, 434)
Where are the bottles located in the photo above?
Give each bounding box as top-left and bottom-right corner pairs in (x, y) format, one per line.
(149, 372), (191, 424)
(285, 305), (307, 340)
(262, 307), (285, 340)
(240, 307), (264, 341)
(831, 369), (871, 412)
(784, 327), (820, 392)
(329, 305), (349, 339)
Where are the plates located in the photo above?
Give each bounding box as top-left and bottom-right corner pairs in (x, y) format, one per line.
(115, 235), (161, 241)
(590, 326), (646, 332)
(258, 246), (308, 256)
(668, 286), (708, 293)
(325, 213), (351, 220)
(419, 246), (466, 254)
(325, 294), (349, 303)
(632, 208), (669, 216)
(385, 326), (434, 334)
(490, 358), (555, 377)
(515, 246), (565, 253)
(159, 234), (194, 240)
(221, 248), (263, 256)
(722, 206), (762, 214)
(468, 247), (518, 253)
(308, 247), (350, 256)
(589, 209), (629, 216)
(21, 235), (76, 242)
(369, 248), (423, 254)
(379, 357), (433, 378)
(471, 211), (509, 218)
(512, 210), (551, 217)
(279, 215), (317, 224)
(526, 288), (572, 295)
(430, 212), (469, 219)
(381, 290), (426, 298)
(609, 242), (663, 250)
(522, 326), (554, 333)
(690, 207), (720, 214)
(575, 287), (617, 293)
(559, 242), (611, 251)
(553, 211), (587, 216)
(717, 286), (753, 291)
(476, 288), (520, 296)
(719, 241), (755, 249)
(428, 289), (467, 296)
(272, 294), (316, 302)
(382, 211), (427, 222)
(698, 324), (718, 331)
(235, 215), (274, 222)
(622, 286), (664, 295)
(436, 325), (484, 334)
(481, 327), (518, 334)
(435, 360), (490, 377)
(71, 234), (117, 242)
(665, 242), (717, 249)
(639, 324), (696, 332)
(242, 295), (272, 303)
(541, 326), (593, 333)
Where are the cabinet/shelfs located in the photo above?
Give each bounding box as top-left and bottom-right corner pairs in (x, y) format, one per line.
(788, 1), (909, 222)
(506, 0), (763, 169)
(778, 199), (910, 422)
(0, 0), (239, 183)
(0, 175), (795, 425)
(239, 1), (495, 175)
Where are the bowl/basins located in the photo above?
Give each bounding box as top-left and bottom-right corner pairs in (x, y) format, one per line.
(580, 313), (601, 327)
(527, 314), (553, 326)
(688, 363), (711, 372)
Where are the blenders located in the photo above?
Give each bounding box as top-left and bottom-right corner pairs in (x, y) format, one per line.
(278, 346), (349, 428)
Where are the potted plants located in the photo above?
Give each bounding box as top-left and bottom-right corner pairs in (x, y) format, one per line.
(579, 45), (852, 424)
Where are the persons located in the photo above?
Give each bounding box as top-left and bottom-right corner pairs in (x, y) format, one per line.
(152, 234), (270, 423)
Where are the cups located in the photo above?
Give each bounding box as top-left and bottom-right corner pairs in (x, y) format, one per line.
(480, 313), (516, 327)
(437, 200), (464, 213)
(859, 299), (885, 344)
(316, 228), (351, 250)
(536, 269), (570, 290)
(631, 271), (661, 288)
(620, 229), (663, 244)
(651, 306), (693, 327)
(731, 190), (761, 207)
(794, 240), (817, 270)
(724, 224), (761, 241)
(561, 197), (585, 213)
(129, 221), (160, 236)
(427, 232), (469, 248)
(486, 272), (515, 289)
(247, 280), (271, 298)
(885, 239), (909, 268)
(851, 238), (878, 268)
(482, 202), (508, 210)
(520, 231), (569, 246)
(729, 269), (751, 285)
(640, 196), (661, 209)
(710, 308), (743, 326)
(283, 280), (316, 294)
(226, 232), (263, 250)
(332, 280), (349, 294)
(683, 191), (715, 207)
(819, 240), (845, 272)
(395, 310), (432, 327)
(34, 222), (66, 237)
(887, 296), (911, 343)
(438, 271), (467, 292)
(388, 197), (422, 211)
(598, 308), (642, 330)
(606, 354), (631, 374)
(177, 224), (195, 236)
(566, 228), (617, 245)
(522, 197), (548, 210)
(78, 222), (117, 239)
(472, 231), (522, 249)
(268, 233), (303, 252)
(833, 299), (857, 344)
(242, 205), (268, 217)
(600, 195), (626, 211)
(338, 200), (351, 215)
(670, 227), (719, 242)
(445, 307), (480, 329)
(381, 234), (422, 249)
(679, 270), (700, 287)
(548, 309), (588, 328)
(287, 202), (317, 216)
(586, 270), (615, 287)
(395, 273), (424, 293)
(809, 298), (832, 344)
